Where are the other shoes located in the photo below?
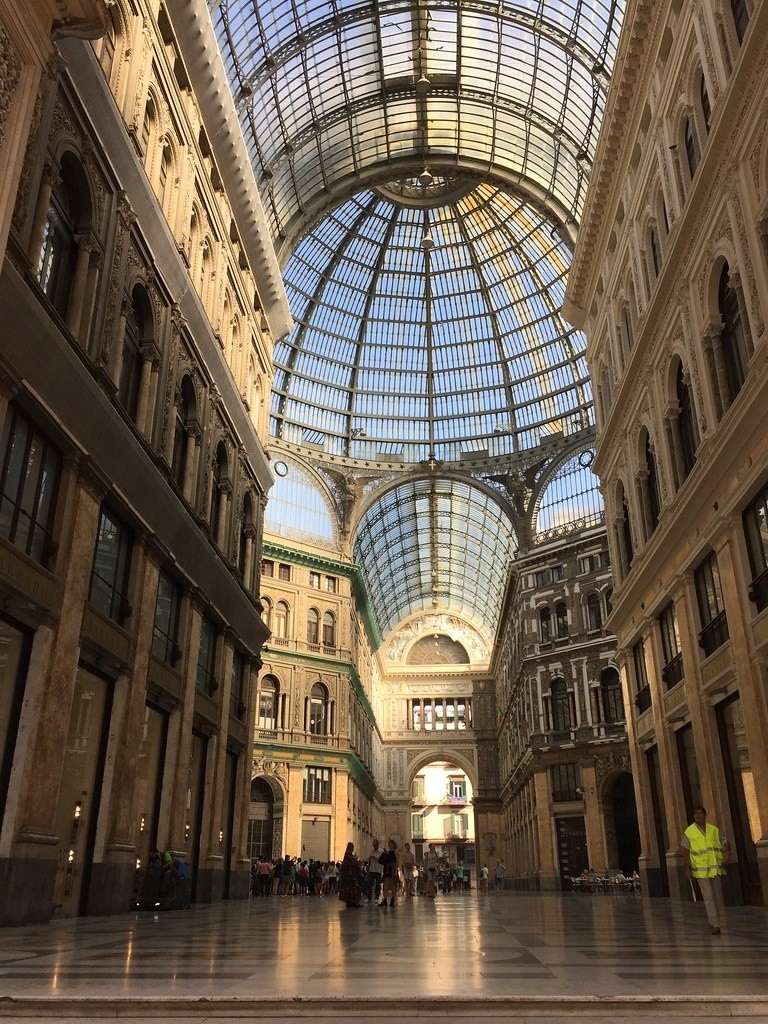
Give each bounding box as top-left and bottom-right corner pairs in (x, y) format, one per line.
(375, 899), (378, 903)
(346, 903), (363, 907)
(712, 927), (721, 935)
(364, 899), (371, 903)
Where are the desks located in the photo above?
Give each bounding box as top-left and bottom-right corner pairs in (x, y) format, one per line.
(626, 878), (635, 893)
(576, 878), (600, 893)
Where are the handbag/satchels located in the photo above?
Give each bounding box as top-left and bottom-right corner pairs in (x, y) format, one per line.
(479, 870), (483, 878)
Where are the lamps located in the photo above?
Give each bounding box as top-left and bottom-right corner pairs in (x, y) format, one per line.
(417, 158), (432, 186)
(415, 73), (433, 95)
(430, 568), (440, 656)
(420, 226), (434, 249)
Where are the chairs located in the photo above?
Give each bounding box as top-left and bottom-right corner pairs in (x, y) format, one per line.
(570, 876), (640, 896)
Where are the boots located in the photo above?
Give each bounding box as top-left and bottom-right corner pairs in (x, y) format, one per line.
(378, 898), (387, 906)
(389, 898), (394, 906)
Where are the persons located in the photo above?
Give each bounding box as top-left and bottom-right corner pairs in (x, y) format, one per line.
(614, 870), (641, 892)
(339, 839), (419, 908)
(149, 848), (172, 897)
(681, 806), (727, 935)
(421, 843), (506, 898)
(251, 853), (343, 898)
(581, 868), (597, 892)
